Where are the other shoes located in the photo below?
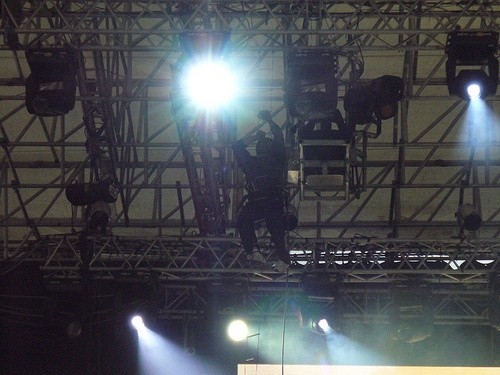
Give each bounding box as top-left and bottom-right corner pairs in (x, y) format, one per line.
(247, 252), (265, 265)
(273, 261), (288, 275)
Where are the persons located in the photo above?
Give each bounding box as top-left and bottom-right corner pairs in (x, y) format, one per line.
(230, 110), (291, 264)
